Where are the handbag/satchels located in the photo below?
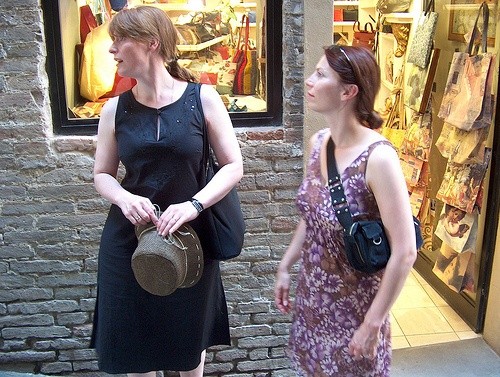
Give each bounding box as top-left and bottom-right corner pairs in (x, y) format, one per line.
(436, 94), (494, 163)
(409, 187), (426, 221)
(400, 111), (434, 162)
(343, 5), (358, 20)
(434, 203), (479, 254)
(80, 20), (119, 101)
(418, 198), (441, 252)
(399, 152), (429, 188)
(353, 21), (375, 52)
(379, 90), (407, 153)
(79, 5), (98, 44)
(169, 2), (259, 95)
(437, 2), (493, 132)
(406, 0), (438, 68)
(334, 9), (344, 21)
(437, 147), (493, 214)
(434, 242), (476, 293)
(344, 215), (423, 273)
(400, 40), (440, 114)
(376, 0), (410, 13)
(195, 84), (246, 260)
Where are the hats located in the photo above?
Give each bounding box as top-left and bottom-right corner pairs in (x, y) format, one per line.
(131, 204), (204, 296)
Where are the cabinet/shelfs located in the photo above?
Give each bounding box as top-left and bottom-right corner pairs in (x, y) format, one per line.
(333, 0), (432, 126)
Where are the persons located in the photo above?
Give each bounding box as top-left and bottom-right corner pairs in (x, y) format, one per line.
(435, 204), (466, 248)
(89, 5), (244, 376)
(276, 45), (416, 377)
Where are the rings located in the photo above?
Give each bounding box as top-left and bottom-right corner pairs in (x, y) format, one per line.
(136, 216), (140, 221)
(360, 355), (363, 358)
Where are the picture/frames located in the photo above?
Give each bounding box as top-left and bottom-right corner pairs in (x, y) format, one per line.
(447, 1), (497, 48)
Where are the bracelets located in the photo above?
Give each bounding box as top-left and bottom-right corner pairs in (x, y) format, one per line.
(188, 196), (204, 213)
